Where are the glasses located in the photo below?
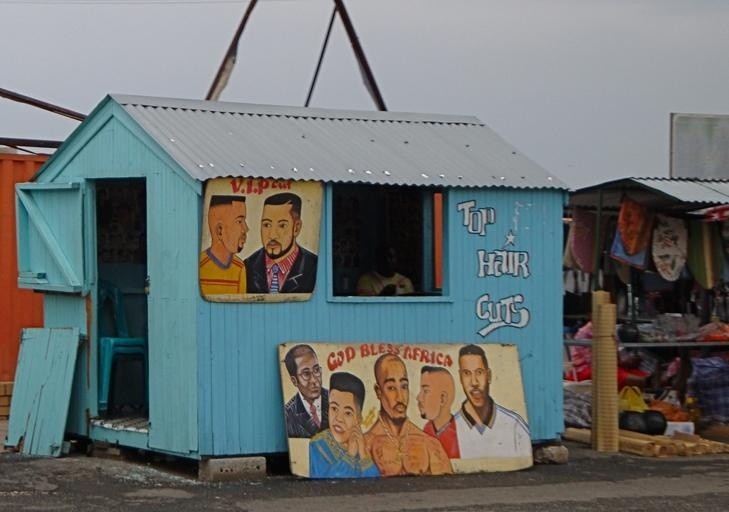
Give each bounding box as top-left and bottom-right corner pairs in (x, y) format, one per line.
(294, 367), (322, 380)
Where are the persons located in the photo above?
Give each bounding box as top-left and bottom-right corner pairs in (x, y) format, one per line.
(571, 322), (653, 392)
(355, 243), (415, 296)
(199, 193), (318, 295)
(284, 345), (533, 479)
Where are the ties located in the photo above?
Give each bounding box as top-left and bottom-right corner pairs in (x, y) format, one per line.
(268, 265), (283, 294)
(309, 404), (322, 428)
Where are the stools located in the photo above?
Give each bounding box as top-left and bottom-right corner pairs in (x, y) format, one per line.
(99, 335), (148, 419)
